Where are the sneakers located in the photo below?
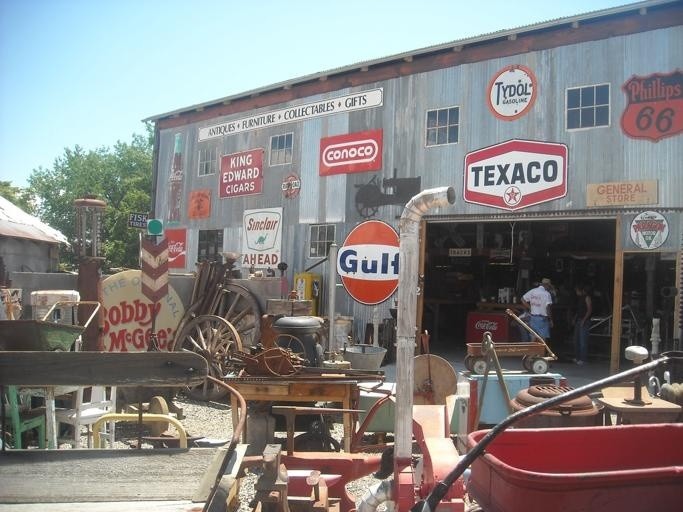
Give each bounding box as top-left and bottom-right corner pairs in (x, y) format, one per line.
(572, 358), (587, 365)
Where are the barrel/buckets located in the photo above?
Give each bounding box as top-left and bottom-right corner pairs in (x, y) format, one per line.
(271, 316), (325, 366)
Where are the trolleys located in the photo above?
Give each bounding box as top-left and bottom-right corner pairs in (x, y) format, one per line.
(464, 309), (558, 374)
(0, 300), (100, 351)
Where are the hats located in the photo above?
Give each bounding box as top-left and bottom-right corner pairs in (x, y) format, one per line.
(539, 279), (553, 287)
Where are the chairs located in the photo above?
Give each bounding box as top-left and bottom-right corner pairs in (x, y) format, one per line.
(54, 385), (118, 451)
(0, 385), (48, 453)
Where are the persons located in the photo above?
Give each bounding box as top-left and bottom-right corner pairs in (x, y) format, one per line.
(510, 275), (593, 366)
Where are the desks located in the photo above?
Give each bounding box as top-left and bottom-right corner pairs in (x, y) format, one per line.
(223, 371), (356, 454)
